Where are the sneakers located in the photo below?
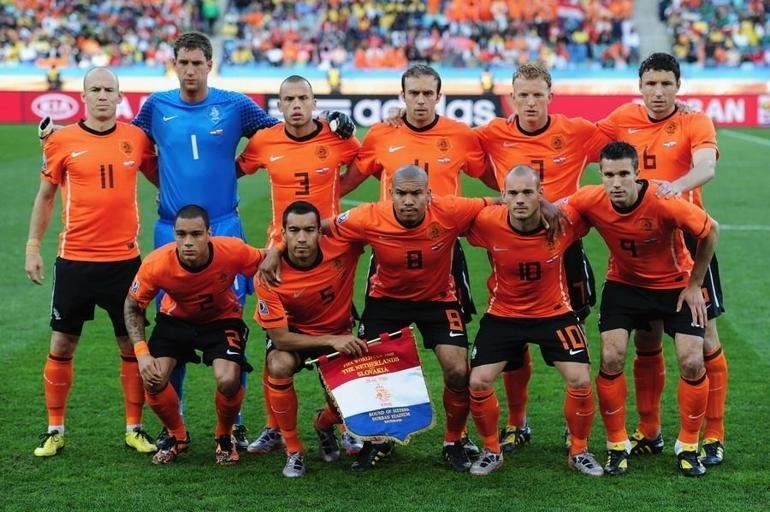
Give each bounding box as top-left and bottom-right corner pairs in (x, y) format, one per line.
(34, 431), (64, 456)
(442, 424), (531, 476)
(314, 408), (395, 471)
(125, 426), (192, 464)
(216, 424), (306, 478)
(564, 424), (724, 478)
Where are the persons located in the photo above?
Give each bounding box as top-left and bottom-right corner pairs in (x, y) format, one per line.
(507, 53), (727, 465)
(340, 65), (500, 457)
(257, 166), (573, 472)
(253, 201), (366, 478)
(383, 64), (614, 456)
(2, 1), (770, 94)
(552, 142), (719, 476)
(457, 166), (606, 477)
(23, 67), (159, 458)
(39, 34), (356, 450)
(234, 75), (382, 456)
(123, 205), (271, 464)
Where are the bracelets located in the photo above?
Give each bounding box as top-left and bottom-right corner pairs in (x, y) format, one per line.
(27, 238), (41, 256)
(132, 339), (149, 359)
